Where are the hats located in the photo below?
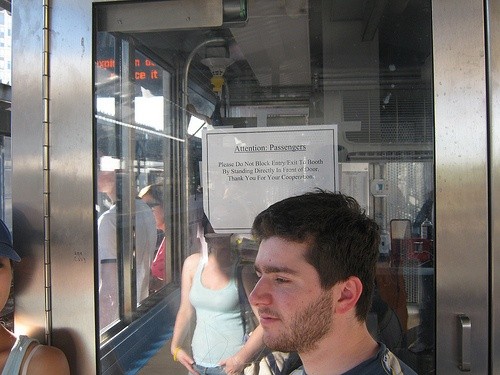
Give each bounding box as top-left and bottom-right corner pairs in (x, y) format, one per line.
(0, 218), (22, 262)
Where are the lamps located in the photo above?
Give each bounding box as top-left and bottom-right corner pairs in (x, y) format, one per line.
(369, 162), (390, 197)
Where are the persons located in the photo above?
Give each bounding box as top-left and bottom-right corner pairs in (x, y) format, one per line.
(136, 182), (167, 286)
(169, 213), (266, 375)
(96, 135), (158, 332)
(247, 187), (418, 375)
(0, 219), (71, 375)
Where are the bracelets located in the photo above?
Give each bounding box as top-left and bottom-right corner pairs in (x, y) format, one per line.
(173, 345), (183, 362)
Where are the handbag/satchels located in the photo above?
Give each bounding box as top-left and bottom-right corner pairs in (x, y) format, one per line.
(236, 262), (299, 375)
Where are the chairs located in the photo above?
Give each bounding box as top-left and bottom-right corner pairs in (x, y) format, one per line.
(386, 218), (425, 267)
